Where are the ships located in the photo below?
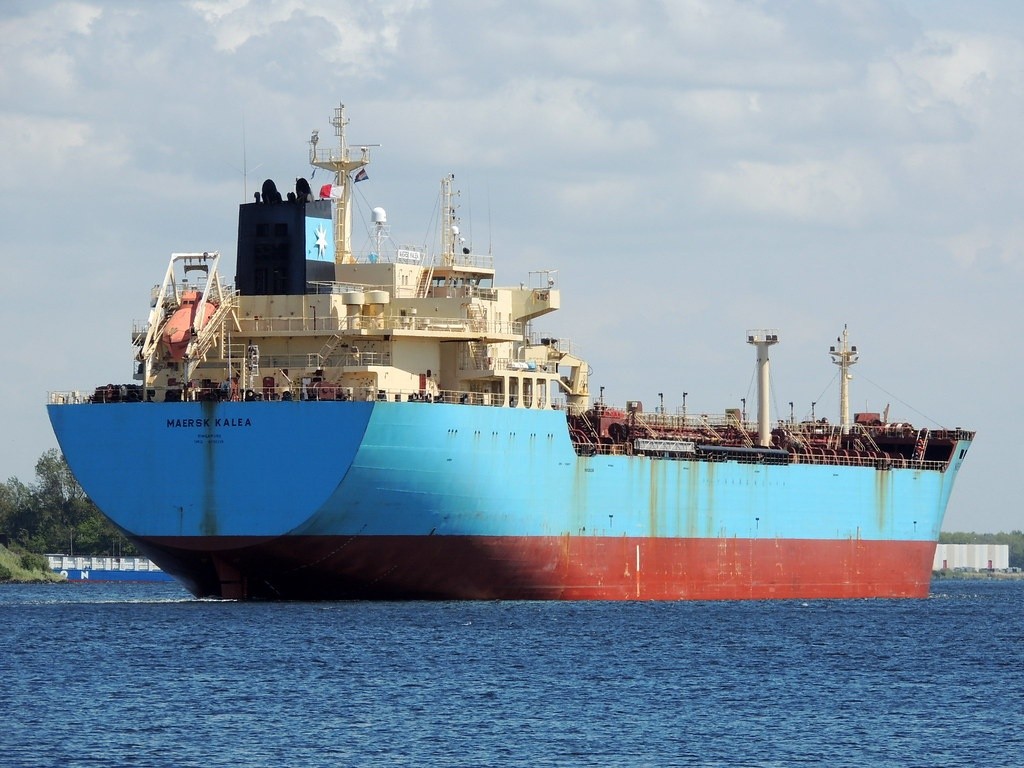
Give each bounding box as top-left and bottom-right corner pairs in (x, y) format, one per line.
(43, 94), (978, 609)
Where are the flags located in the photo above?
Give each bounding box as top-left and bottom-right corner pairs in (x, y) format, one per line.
(352, 168), (369, 183)
(319, 184), (344, 199)
